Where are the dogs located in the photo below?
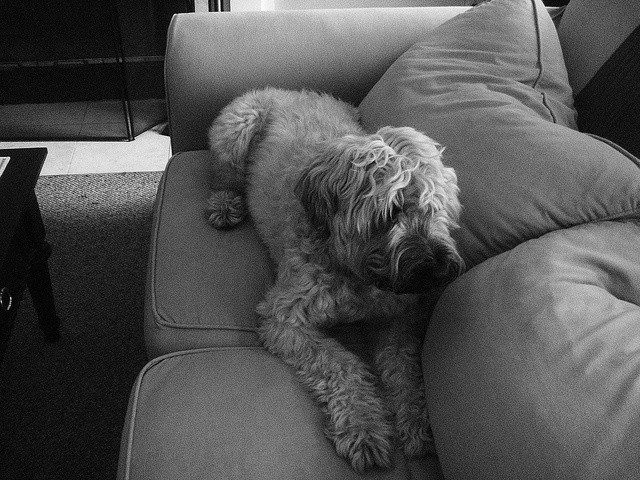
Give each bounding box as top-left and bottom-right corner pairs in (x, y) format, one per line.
(202, 84), (469, 476)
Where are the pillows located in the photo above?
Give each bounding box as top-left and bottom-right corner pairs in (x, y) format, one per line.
(355, 0), (640, 270)
(421, 219), (640, 478)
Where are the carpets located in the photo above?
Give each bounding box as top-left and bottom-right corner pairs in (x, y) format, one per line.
(0, 172), (168, 480)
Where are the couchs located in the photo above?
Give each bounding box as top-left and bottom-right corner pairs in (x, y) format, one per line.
(120, 1), (640, 480)
